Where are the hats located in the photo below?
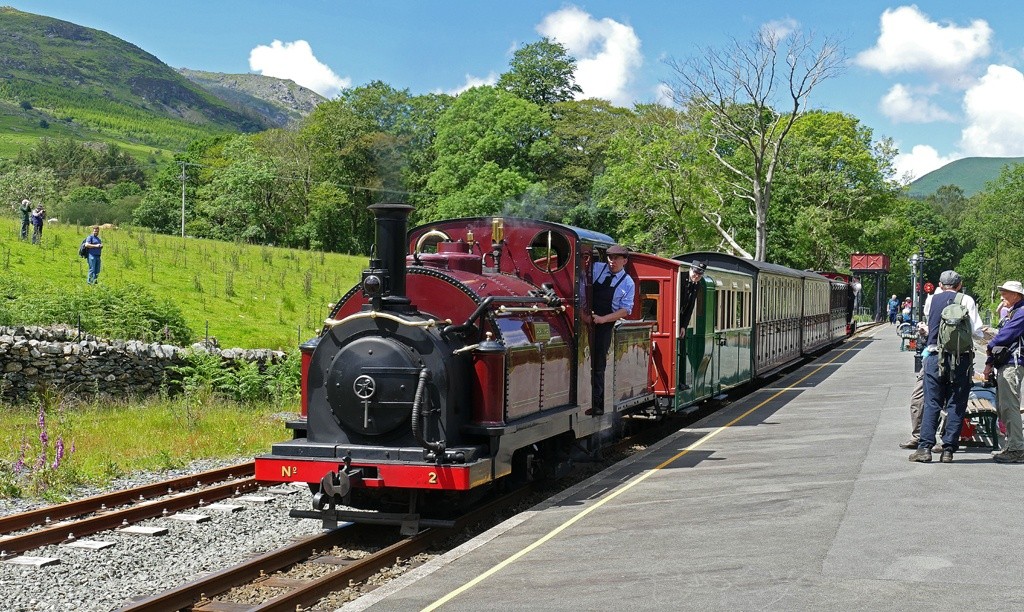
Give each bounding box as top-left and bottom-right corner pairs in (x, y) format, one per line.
(997, 281), (1024, 296)
(939, 270), (962, 285)
(35, 205), (43, 210)
(606, 245), (629, 255)
(690, 260), (707, 274)
(905, 296), (910, 300)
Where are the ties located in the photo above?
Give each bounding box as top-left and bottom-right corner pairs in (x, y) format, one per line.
(687, 282), (694, 296)
(602, 274), (616, 287)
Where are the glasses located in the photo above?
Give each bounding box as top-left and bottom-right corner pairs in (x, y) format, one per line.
(691, 268), (703, 277)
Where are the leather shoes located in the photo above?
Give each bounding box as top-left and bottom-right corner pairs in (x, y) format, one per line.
(679, 382), (689, 391)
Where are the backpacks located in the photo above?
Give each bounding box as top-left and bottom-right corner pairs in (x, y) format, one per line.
(936, 291), (974, 383)
(79, 235), (93, 259)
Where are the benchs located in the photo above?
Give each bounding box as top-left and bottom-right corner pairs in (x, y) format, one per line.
(958, 374), (1002, 452)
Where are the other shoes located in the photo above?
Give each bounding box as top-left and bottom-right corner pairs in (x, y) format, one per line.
(899, 439), (919, 449)
(592, 409), (603, 416)
(930, 443), (943, 453)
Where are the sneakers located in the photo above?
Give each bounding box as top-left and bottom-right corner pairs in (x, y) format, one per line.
(909, 445), (931, 462)
(991, 445), (1024, 463)
(940, 450), (952, 463)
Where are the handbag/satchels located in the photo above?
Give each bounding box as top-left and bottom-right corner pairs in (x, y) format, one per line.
(991, 346), (1011, 364)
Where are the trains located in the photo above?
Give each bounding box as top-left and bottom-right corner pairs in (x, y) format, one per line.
(254, 203), (861, 541)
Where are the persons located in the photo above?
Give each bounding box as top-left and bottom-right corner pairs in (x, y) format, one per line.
(847, 276), (862, 322)
(887, 295), (900, 326)
(899, 270), (988, 463)
(996, 296), (1012, 325)
(983, 281), (1024, 463)
(85, 227), (104, 285)
(648, 260), (707, 391)
(19, 199), (31, 241)
(901, 296), (912, 321)
(579, 244), (592, 324)
(593, 246), (635, 416)
(31, 204), (45, 245)
